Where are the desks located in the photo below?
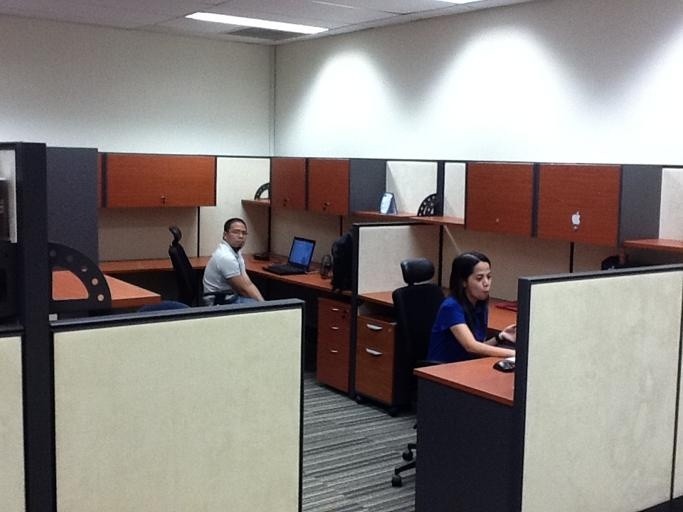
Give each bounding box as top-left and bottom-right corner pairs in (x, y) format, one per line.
(360, 281), (516, 512)
(51, 250), (337, 306)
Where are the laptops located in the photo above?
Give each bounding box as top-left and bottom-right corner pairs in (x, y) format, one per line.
(264, 236), (316, 277)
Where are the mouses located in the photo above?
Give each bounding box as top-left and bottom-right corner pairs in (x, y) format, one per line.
(493, 359), (515, 373)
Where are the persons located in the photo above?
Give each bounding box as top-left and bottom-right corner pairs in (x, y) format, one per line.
(426, 250), (517, 366)
(202, 217), (266, 307)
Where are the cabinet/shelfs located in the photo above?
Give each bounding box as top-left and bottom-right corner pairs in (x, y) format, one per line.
(306, 158), (385, 216)
(349, 159), (440, 218)
(270, 158), (308, 212)
(535, 163), (682, 252)
(312, 292), (395, 409)
(241, 157), (270, 204)
(101, 153), (217, 208)
(410, 161), (535, 238)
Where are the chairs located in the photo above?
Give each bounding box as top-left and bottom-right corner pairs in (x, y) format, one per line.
(167, 224), (240, 308)
(391, 257), (447, 486)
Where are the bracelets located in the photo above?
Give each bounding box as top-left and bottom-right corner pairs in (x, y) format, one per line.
(495, 333), (504, 345)
